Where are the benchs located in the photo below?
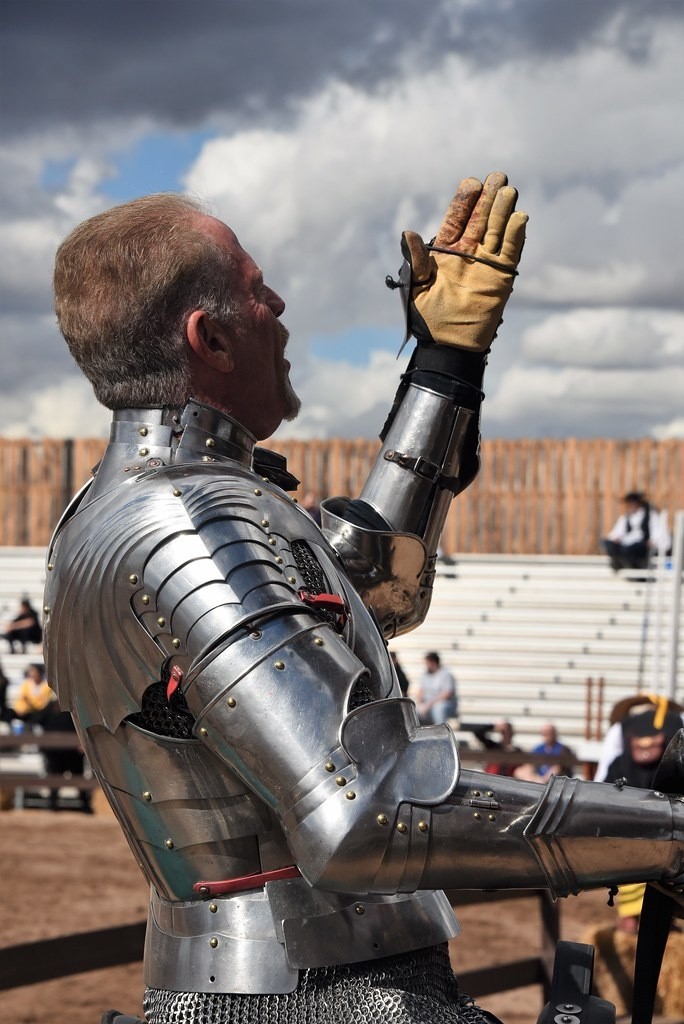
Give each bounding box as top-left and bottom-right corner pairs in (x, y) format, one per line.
(0, 546), (684, 811)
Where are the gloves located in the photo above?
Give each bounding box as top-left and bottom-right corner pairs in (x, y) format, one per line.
(381, 170), (531, 493)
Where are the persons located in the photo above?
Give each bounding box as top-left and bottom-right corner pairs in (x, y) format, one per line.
(0, 601), (40, 653)
(483, 723), (573, 783)
(42, 173), (532, 1024)
(0, 664), (94, 815)
(601, 493), (670, 583)
(603, 709), (684, 933)
(414, 652), (457, 726)
